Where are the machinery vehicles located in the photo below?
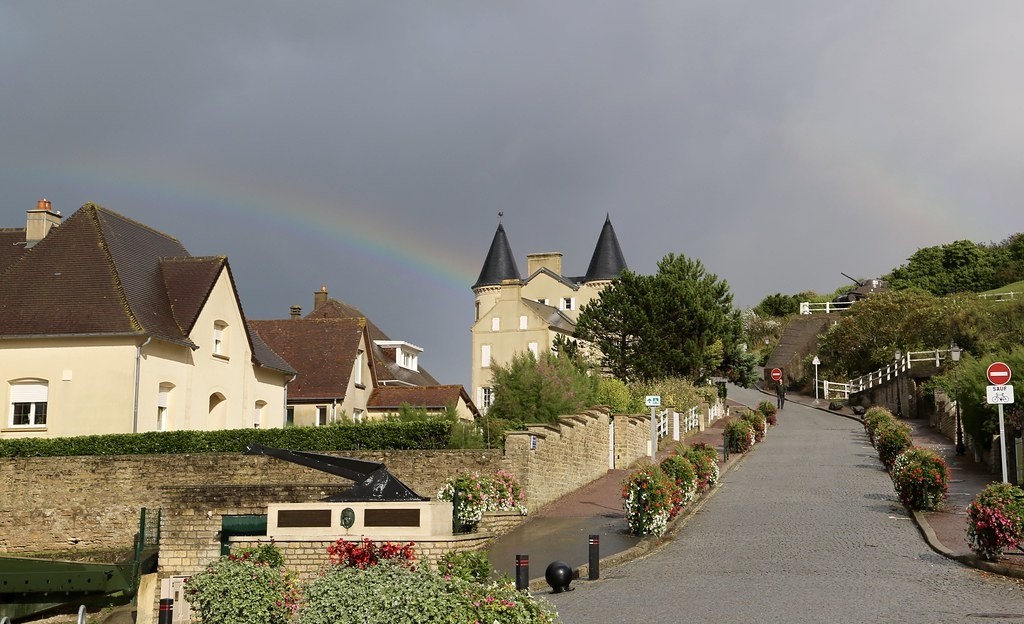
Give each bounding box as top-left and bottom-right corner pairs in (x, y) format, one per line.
(833, 271), (892, 310)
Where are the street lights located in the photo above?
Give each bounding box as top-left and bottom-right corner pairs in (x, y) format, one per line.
(894, 348), (903, 417)
(950, 342), (969, 456)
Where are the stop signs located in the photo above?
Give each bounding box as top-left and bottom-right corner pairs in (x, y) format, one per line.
(771, 367), (782, 381)
(987, 363), (1011, 385)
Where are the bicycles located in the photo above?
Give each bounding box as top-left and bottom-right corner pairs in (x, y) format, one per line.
(992, 393), (1009, 402)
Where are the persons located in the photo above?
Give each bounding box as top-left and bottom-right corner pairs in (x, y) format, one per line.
(776, 379), (787, 410)
